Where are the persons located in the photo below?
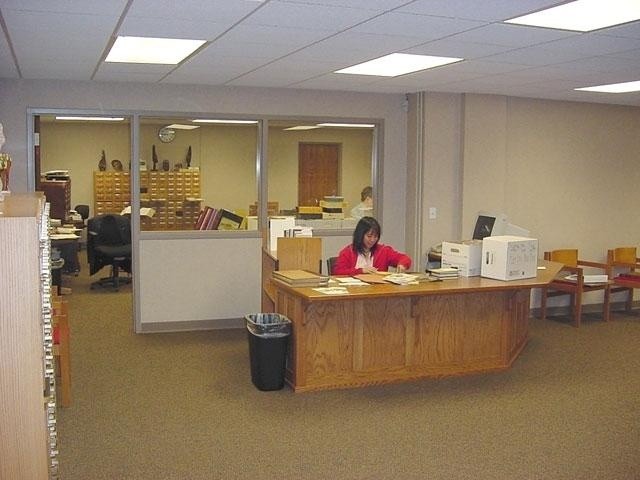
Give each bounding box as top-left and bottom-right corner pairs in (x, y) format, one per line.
(350, 186), (372, 218)
(335, 216), (411, 277)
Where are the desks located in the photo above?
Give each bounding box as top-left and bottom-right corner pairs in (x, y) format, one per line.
(272, 257), (566, 395)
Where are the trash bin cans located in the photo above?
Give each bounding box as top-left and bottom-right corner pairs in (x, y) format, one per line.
(52, 258), (64, 295)
(245, 313), (292, 391)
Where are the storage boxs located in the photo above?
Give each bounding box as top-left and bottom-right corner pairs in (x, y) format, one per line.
(440, 239), (481, 278)
(480, 235), (539, 281)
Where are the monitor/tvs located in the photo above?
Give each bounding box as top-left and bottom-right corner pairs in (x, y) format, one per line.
(471, 211), (530, 242)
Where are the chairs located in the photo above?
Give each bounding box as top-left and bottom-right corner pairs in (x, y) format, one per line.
(71, 204), (90, 251)
(50, 285), (72, 407)
(542, 247), (615, 327)
(87, 214), (133, 292)
(601, 246), (640, 321)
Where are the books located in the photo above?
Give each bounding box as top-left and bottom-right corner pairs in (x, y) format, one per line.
(426, 268), (458, 281)
(272, 269), (330, 283)
(274, 276), (327, 288)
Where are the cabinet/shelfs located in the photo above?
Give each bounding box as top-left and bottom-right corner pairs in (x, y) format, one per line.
(40, 175), (71, 220)
(0, 189), (61, 478)
(92, 167), (204, 230)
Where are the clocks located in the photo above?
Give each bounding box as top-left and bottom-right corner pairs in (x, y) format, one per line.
(157, 124), (177, 144)
